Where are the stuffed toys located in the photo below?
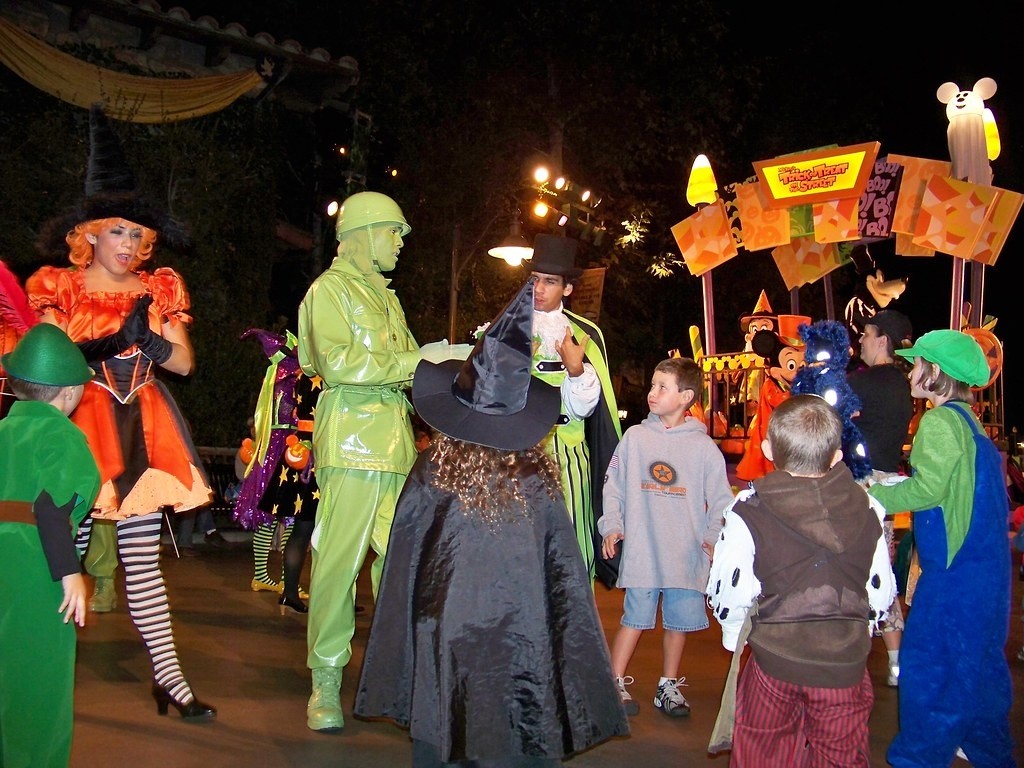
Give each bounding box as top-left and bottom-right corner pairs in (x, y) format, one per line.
(733, 244), (909, 479)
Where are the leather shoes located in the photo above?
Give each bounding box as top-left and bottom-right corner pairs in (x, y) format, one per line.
(250, 578), (278, 592)
(277, 581), (310, 600)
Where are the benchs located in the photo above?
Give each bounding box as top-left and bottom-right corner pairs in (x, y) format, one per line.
(163, 458), (236, 559)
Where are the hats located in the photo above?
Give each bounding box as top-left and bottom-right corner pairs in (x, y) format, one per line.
(412, 276), (562, 452)
(240, 328), (301, 372)
(35, 104), (194, 263)
(521, 233), (584, 278)
(0, 322), (96, 386)
(856, 309), (911, 351)
(895, 329), (991, 387)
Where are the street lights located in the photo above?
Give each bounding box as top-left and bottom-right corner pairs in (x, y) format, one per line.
(529, 161), (615, 249)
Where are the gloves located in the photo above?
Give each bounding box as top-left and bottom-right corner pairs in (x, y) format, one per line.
(73, 292), (173, 364)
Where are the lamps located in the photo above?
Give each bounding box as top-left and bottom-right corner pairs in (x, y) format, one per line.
(571, 183), (606, 248)
(549, 208), (568, 229)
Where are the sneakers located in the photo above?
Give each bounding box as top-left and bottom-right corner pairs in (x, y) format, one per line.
(653, 676), (690, 716)
(614, 673), (639, 715)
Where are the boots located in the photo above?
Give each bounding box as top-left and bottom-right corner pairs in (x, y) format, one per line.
(307, 666), (343, 735)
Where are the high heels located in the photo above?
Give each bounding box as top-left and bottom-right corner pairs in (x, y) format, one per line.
(278, 594), (309, 616)
(152, 677), (218, 723)
(354, 604), (365, 611)
(87, 579), (118, 611)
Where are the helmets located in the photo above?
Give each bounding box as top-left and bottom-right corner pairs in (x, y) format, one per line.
(336, 191), (412, 243)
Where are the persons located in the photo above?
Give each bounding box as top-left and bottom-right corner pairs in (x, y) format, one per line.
(865, 327), (1013, 768)
(840, 309), (915, 689)
(298, 191), (477, 735)
(350, 275), (635, 768)
(471, 231), (623, 600)
(704, 393), (900, 768)
(597, 356), (736, 721)
(177, 327), (364, 618)
(22, 100), (217, 724)
(0, 262), (104, 768)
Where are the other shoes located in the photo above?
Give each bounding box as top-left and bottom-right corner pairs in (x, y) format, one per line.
(204, 530), (229, 547)
(172, 547), (202, 557)
(887, 661), (901, 686)
